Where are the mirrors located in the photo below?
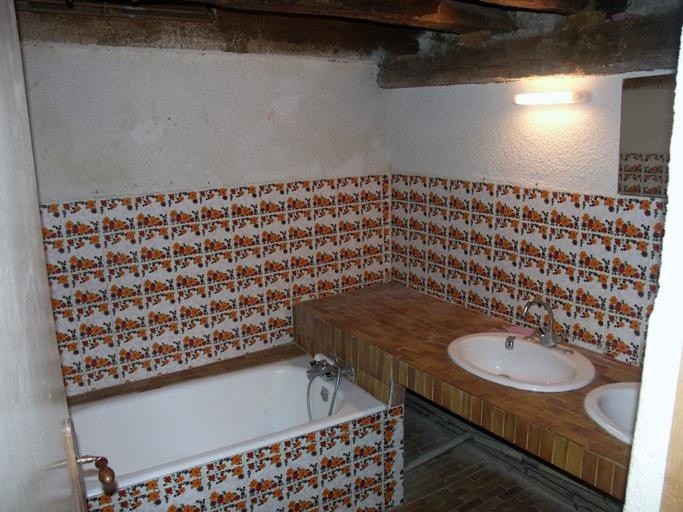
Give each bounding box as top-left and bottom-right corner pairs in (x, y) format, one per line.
(617, 72), (676, 198)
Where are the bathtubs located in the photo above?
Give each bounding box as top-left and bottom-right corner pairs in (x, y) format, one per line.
(68, 355), (387, 499)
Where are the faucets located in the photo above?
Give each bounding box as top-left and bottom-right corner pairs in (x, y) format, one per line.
(522, 301), (554, 332)
(306, 359), (336, 379)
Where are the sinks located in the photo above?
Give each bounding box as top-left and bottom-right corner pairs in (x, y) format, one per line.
(585, 381), (645, 445)
(448, 331), (596, 393)
